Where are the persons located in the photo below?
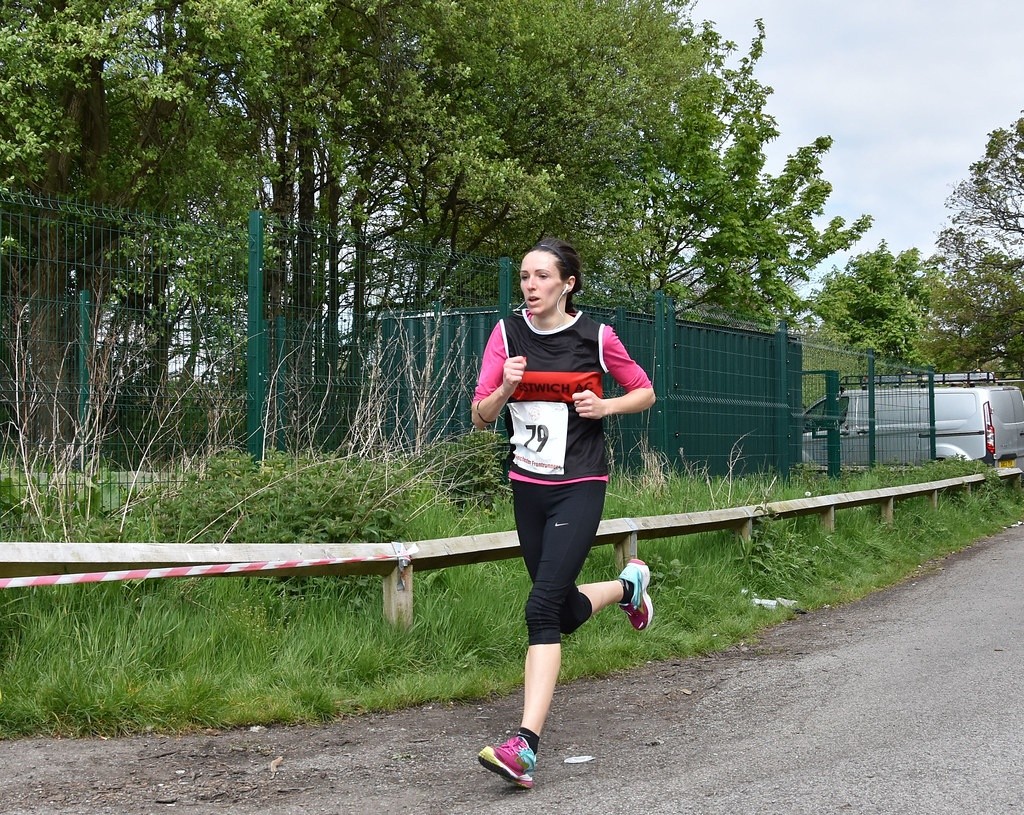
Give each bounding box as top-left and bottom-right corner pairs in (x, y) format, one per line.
(472, 239), (656, 790)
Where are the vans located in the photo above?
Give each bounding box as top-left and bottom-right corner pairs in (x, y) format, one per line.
(803, 374), (1024, 469)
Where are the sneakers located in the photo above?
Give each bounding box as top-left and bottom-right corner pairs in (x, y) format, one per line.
(619, 558), (653, 631)
(478, 736), (536, 789)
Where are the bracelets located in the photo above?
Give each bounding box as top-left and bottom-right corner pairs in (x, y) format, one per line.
(475, 401), (499, 425)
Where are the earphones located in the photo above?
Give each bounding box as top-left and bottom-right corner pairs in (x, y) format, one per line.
(562, 283), (569, 295)
